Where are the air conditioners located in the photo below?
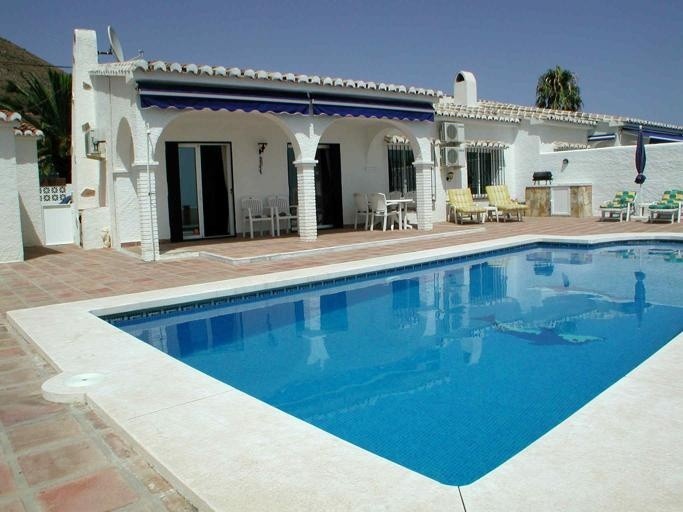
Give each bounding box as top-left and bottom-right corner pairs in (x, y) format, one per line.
(440, 121), (465, 143)
(440, 147), (465, 167)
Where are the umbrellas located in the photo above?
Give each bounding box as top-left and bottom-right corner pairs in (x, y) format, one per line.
(633, 249), (647, 328)
(633, 125), (647, 215)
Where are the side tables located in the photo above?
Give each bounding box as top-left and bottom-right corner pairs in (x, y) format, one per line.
(629, 202), (650, 222)
(481, 206), (498, 224)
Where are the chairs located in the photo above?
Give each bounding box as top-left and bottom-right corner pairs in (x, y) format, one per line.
(647, 186), (682, 224)
(446, 187), (487, 224)
(240, 195), (274, 239)
(389, 191), (402, 230)
(370, 192), (401, 231)
(267, 194), (297, 237)
(484, 186), (527, 220)
(599, 190), (635, 223)
(353, 193), (383, 230)
